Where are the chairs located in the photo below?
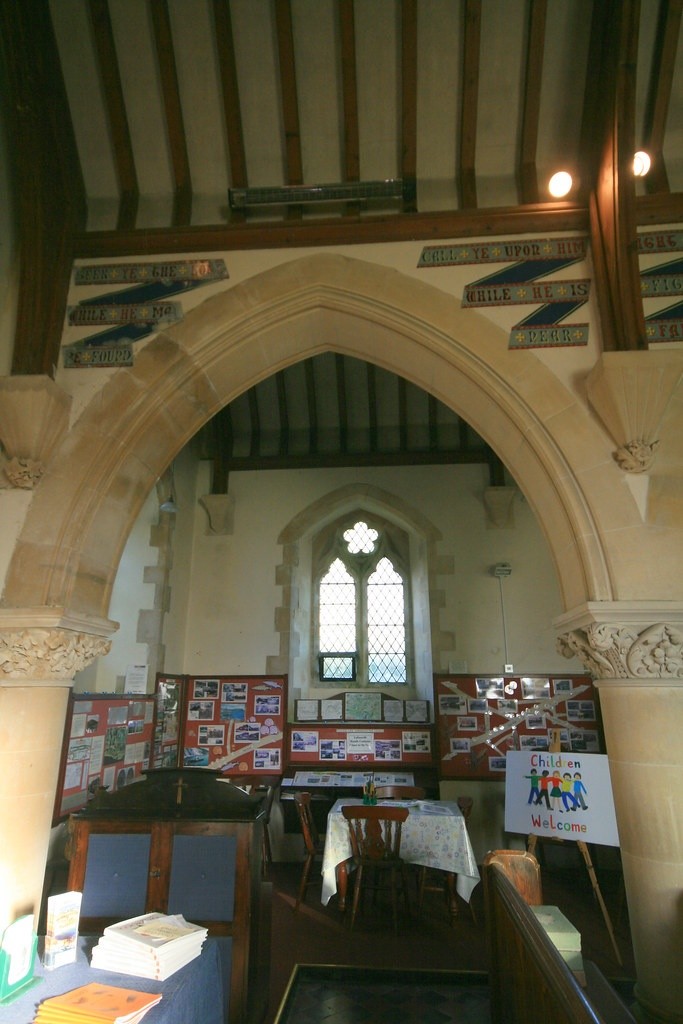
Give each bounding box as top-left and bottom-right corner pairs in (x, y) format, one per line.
(374, 785), (426, 801)
(417, 794), (475, 920)
(342, 804), (410, 935)
(296, 792), (326, 908)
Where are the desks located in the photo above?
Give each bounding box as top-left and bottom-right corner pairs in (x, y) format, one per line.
(321, 798), (481, 925)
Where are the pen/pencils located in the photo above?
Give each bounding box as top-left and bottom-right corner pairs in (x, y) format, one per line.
(364, 777), (376, 804)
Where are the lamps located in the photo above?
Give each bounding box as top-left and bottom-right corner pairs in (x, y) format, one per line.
(493, 562), (511, 577)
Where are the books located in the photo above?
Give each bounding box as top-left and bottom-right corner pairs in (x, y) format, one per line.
(90, 912), (208, 982)
(42, 891), (82, 970)
(33, 983), (163, 1024)
